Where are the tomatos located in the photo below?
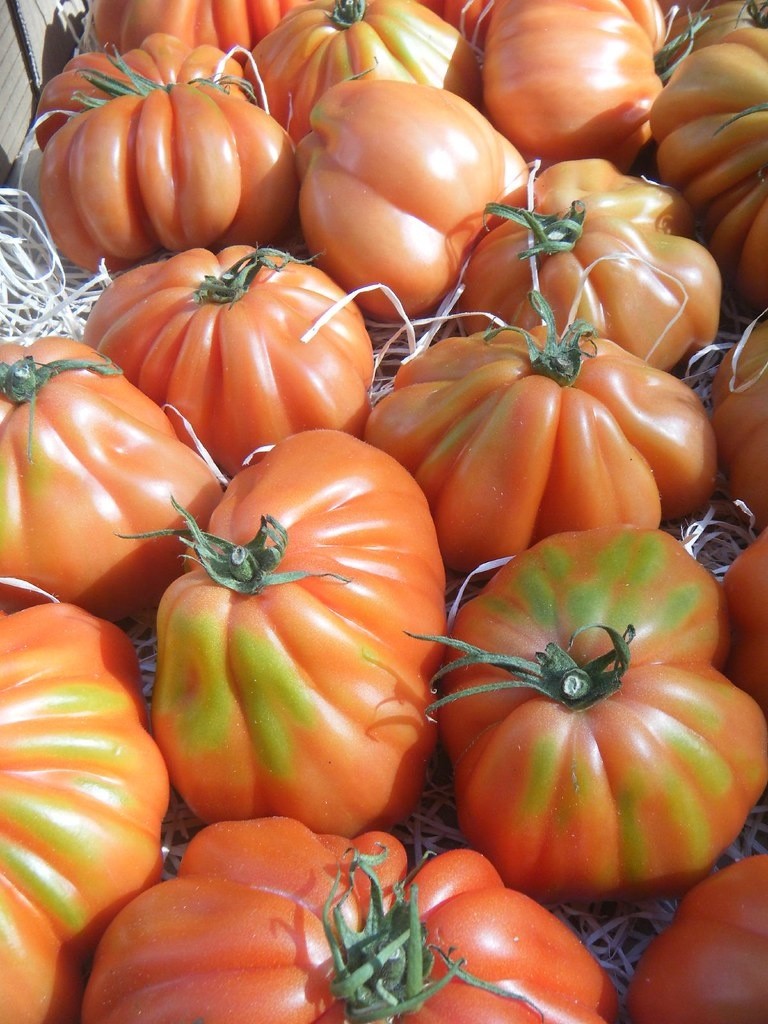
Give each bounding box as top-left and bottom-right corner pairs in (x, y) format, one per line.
(0, 0), (768, 1022)
